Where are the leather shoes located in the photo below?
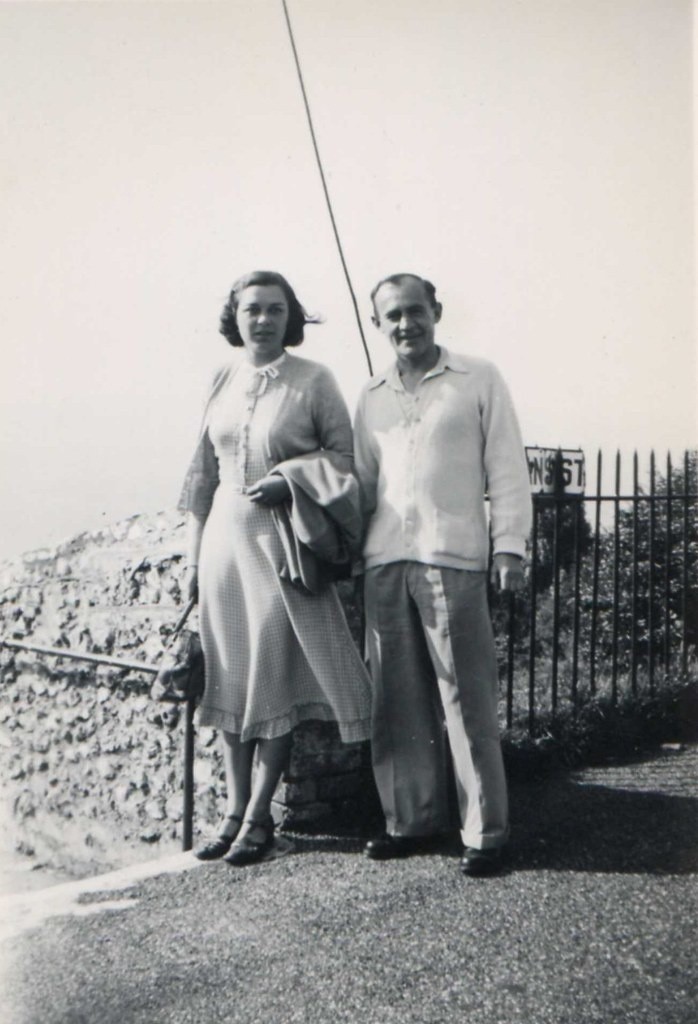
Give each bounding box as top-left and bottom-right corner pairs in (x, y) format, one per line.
(459, 844), (509, 873)
(364, 832), (426, 858)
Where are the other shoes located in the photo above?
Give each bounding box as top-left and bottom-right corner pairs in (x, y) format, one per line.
(192, 812), (246, 859)
(223, 814), (276, 862)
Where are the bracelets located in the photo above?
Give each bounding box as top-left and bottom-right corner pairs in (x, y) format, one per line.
(182, 563), (197, 571)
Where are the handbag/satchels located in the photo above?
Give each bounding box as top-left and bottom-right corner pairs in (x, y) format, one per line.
(150, 595), (204, 700)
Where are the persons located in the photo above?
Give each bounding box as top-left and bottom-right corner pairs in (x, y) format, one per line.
(176, 271), (373, 866)
(353, 274), (534, 874)
